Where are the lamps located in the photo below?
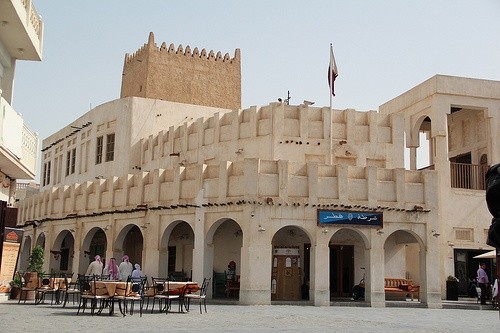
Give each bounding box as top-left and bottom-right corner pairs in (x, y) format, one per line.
(448, 240), (455, 248)
(24, 233), (34, 240)
(258, 225), (266, 232)
(432, 230), (440, 238)
(40, 230), (49, 239)
(103, 224), (113, 232)
(69, 226), (79, 233)
(376, 228), (384, 236)
(140, 222), (151, 230)
(320, 225), (330, 234)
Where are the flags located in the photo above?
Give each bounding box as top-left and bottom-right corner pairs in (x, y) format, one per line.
(328, 46), (338, 96)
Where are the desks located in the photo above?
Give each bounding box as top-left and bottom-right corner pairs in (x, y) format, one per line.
(147, 281), (198, 313)
(37, 277), (72, 306)
(82, 280), (140, 316)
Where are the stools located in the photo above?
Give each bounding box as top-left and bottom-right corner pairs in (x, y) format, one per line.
(410, 285), (420, 302)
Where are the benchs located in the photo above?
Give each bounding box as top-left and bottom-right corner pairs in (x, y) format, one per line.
(359, 278), (408, 302)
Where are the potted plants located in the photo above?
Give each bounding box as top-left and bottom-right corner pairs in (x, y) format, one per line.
(9, 244), (47, 300)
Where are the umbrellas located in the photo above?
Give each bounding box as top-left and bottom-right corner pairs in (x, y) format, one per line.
(472, 250), (496, 259)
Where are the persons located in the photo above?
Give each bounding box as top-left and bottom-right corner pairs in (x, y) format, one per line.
(477, 261), (489, 305)
(80, 254), (143, 309)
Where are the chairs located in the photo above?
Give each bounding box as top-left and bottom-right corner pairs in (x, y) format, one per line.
(17, 270), (241, 318)
(473, 284), (494, 304)
